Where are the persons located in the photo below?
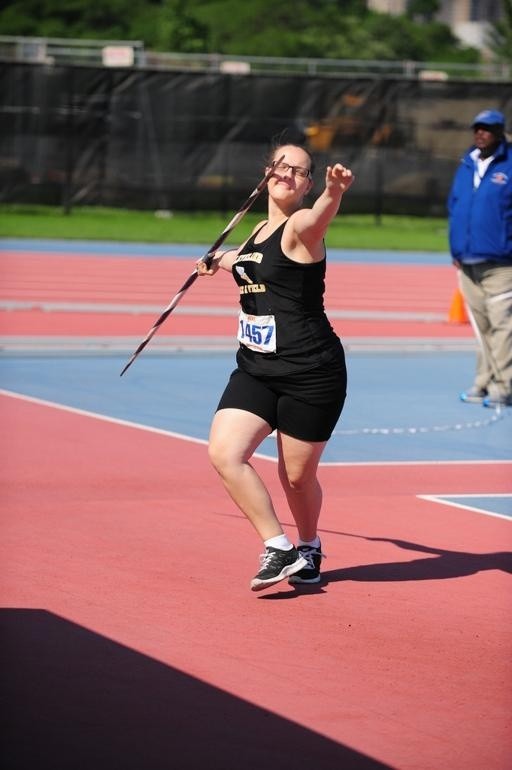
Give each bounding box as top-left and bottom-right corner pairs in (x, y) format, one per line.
(194, 142), (354, 591)
(448, 109), (512, 406)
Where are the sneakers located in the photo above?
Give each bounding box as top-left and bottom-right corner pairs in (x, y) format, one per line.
(250, 541), (308, 592)
(287, 536), (327, 584)
(459, 383), (511, 407)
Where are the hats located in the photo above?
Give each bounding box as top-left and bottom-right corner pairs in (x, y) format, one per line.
(469, 108), (505, 130)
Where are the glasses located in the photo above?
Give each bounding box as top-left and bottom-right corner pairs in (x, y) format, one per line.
(267, 161), (312, 181)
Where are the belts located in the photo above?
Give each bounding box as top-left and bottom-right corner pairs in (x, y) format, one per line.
(459, 259), (503, 273)
